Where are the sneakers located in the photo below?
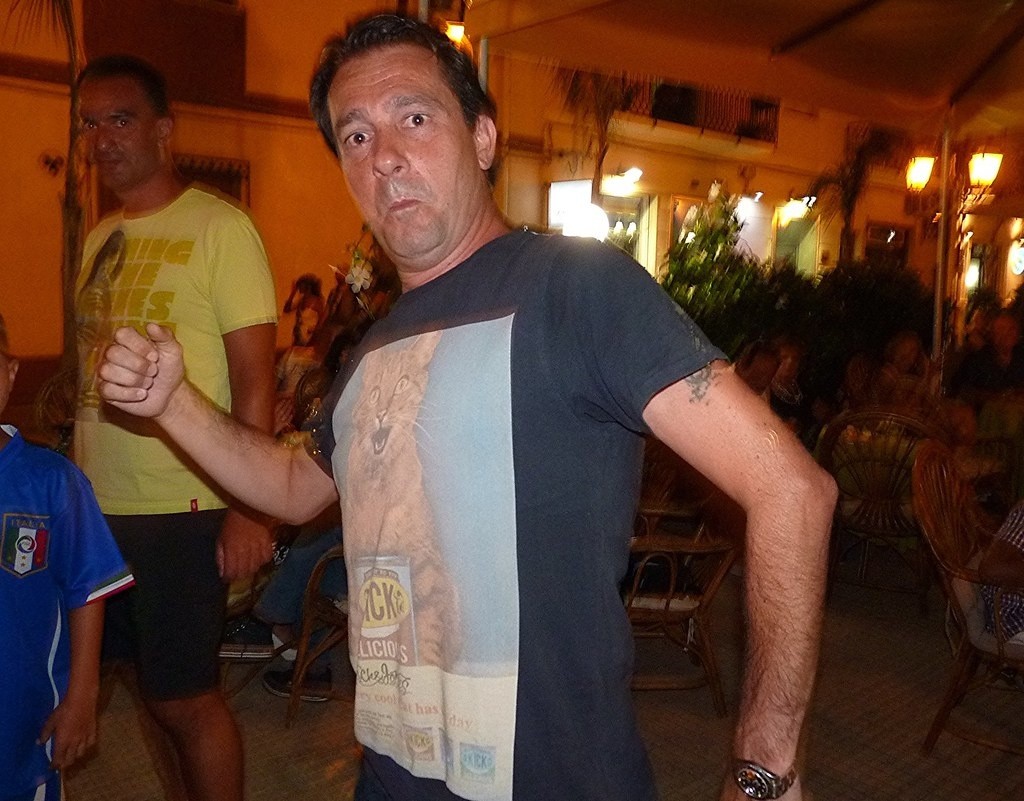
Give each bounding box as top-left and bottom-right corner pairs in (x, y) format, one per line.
(261, 660), (336, 701)
(217, 614), (275, 658)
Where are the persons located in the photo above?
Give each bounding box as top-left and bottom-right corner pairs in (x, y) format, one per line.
(219, 232), (1024, 704)
(0, 318), (135, 801)
(98, 9), (838, 801)
(75, 52), (278, 801)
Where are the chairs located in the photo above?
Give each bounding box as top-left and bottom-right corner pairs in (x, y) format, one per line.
(218, 360), (1024, 762)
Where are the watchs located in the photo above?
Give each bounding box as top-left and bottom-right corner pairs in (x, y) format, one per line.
(725, 747), (800, 801)
